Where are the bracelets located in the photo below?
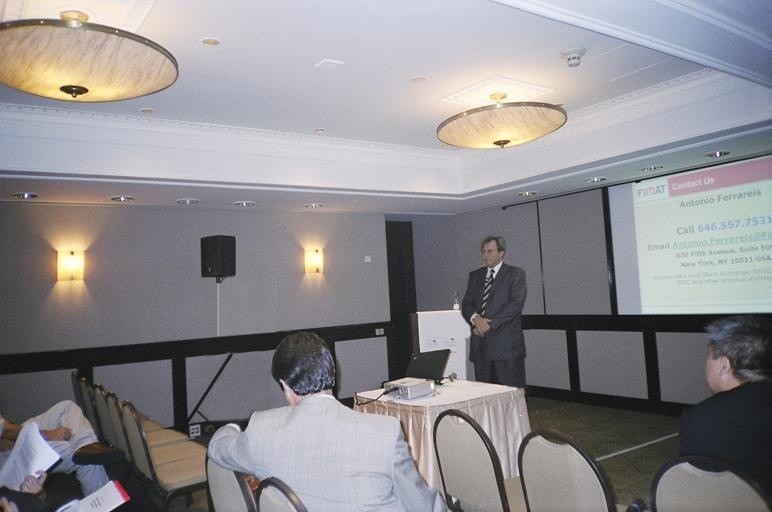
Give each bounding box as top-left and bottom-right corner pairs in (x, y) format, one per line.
(471, 316), (480, 321)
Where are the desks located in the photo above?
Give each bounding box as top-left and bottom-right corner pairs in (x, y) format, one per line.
(353, 376), (534, 511)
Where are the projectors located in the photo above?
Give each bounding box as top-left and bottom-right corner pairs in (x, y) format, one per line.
(384, 377), (435, 399)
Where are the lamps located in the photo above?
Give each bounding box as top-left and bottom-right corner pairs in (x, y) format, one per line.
(302, 246), (326, 275)
(435, 91), (570, 151)
(0, 8), (180, 105)
(55, 245), (87, 282)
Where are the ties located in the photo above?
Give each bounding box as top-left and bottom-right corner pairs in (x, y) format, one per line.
(481, 269), (495, 318)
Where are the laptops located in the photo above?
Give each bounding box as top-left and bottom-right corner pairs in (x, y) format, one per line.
(378, 349), (451, 385)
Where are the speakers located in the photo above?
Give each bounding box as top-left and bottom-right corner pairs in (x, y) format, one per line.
(200, 235), (236, 276)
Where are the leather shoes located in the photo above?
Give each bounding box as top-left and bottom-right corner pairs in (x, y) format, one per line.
(71, 442), (124, 465)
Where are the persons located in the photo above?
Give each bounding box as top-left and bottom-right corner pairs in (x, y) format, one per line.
(679, 316), (771, 510)
(1, 470), (47, 511)
(1, 400), (126, 498)
(462, 235), (526, 389)
(206, 331), (454, 512)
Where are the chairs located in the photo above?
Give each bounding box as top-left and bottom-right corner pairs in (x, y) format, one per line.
(432, 408), (511, 511)
(651, 454), (770, 511)
(66, 368), (309, 512)
(517, 427), (615, 511)
